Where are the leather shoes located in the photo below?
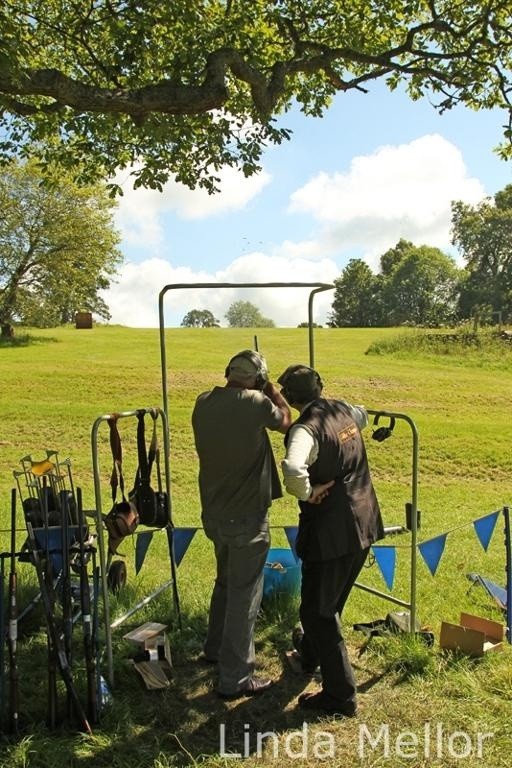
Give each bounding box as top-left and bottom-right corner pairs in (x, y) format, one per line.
(217, 678), (273, 698)
(293, 627), (317, 671)
(298, 692), (357, 715)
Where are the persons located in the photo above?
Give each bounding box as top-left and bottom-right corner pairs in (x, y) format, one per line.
(278, 366), (385, 716)
(192, 350), (291, 695)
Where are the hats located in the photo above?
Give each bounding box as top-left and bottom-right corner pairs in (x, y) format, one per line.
(227, 350), (269, 378)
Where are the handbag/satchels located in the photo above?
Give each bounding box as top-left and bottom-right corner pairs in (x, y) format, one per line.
(105, 486), (169, 539)
(385, 611), (434, 649)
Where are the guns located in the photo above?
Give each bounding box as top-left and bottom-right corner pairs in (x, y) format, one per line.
(5, 478), (101, 738)
(254, 336), (259, 352)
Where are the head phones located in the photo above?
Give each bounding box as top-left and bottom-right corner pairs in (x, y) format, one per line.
(225, 352), (268, 390)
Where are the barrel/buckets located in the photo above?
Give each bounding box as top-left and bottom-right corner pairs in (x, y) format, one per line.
(262, 548), (304, 611)
(74, 311), (92, 329)
